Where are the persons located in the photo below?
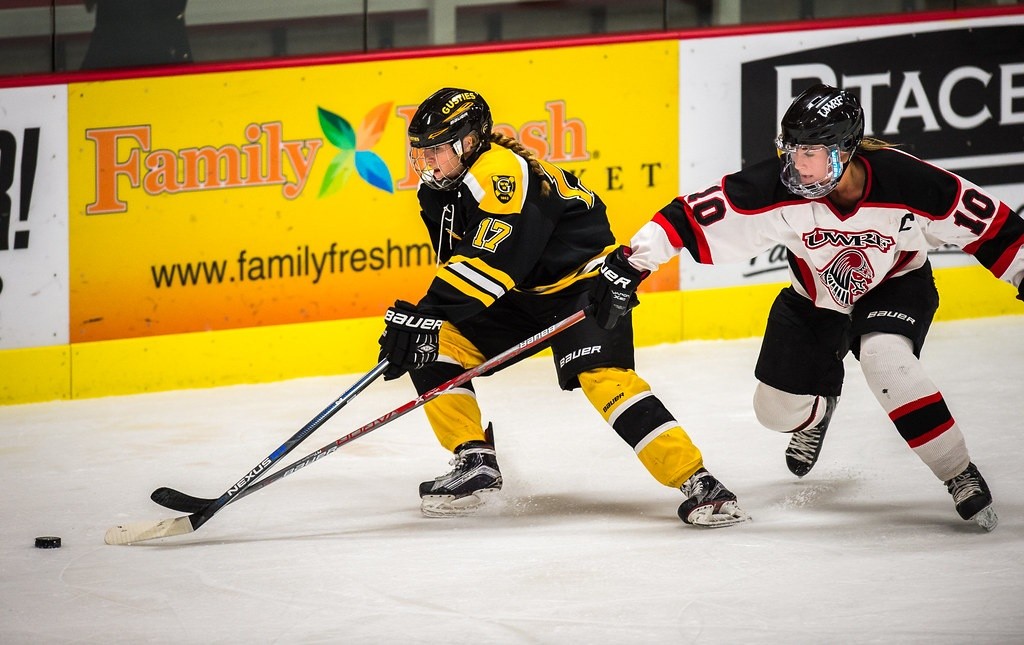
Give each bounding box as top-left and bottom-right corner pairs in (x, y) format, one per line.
(376, 85), (740, 523)
(593, 83), (1024, 520)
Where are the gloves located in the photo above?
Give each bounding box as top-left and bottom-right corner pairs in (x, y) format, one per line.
(588, 244), (652, 331)
(377, 299), (443, 381)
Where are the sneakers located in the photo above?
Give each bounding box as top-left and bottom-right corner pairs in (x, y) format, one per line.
(785, 397), (838, 479)
(421, 422), (503, 518)
(943, 463), (1000, 532)
(677, 467), (753, 528)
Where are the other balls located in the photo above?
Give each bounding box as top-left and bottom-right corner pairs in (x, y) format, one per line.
(34, 536), (62, 549)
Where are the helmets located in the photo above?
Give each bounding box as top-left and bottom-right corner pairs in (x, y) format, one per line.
(775, 84), (865, 200)
(405, 88), (493, 192)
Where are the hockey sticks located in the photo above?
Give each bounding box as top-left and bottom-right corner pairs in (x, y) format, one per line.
(100, 354), (393, 550)
(149, 291), (639, 515)
